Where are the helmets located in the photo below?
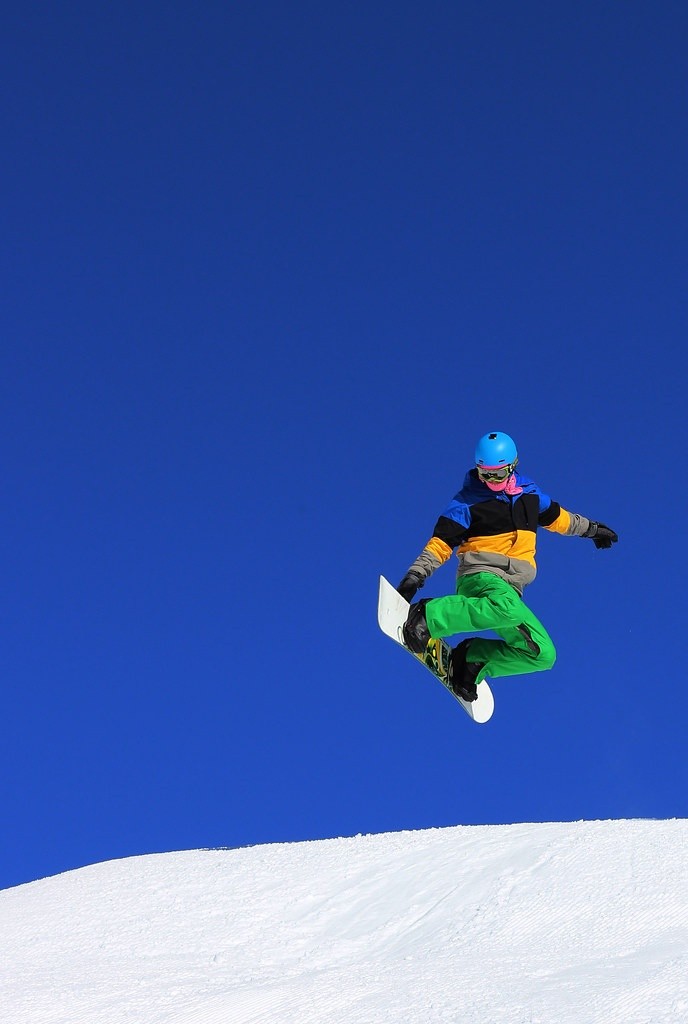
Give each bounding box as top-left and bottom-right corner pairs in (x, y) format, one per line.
(473, 431), (518, 483)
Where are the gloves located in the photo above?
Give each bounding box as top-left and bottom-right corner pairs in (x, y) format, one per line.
(394, 571), (425, 604)
(580, 520), (618, 550)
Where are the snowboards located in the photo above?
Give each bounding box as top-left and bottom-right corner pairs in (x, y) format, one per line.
(377, 573), (495, 726)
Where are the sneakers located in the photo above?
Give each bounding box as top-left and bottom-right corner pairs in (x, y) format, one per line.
(451, 637), (485, 702)
(402, 597), (431, 653)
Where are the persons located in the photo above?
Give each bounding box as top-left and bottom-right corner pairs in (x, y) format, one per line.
(397, 430), (618, 702)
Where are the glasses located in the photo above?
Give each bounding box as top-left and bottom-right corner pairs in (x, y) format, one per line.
(477, 468), (508, 480)
